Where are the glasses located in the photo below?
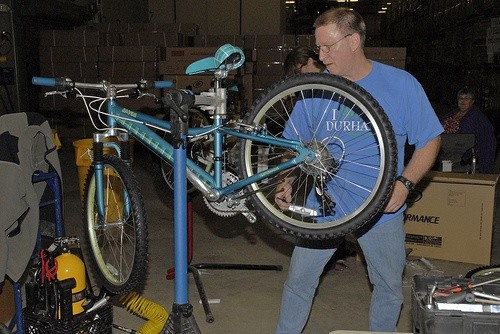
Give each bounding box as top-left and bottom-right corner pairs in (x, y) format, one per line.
(294, 64), (304, 74)
(457, 96), (476, 100)
(313, 34), (352, 54)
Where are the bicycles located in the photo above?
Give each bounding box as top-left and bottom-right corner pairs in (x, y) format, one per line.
(31, 43), (398, 297)
(402, 247), (500, 279)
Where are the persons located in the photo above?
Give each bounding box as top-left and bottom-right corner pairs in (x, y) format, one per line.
(264, 46), (325, 147)
(274, 9), (444, 334)
(437, 86), (498, 173)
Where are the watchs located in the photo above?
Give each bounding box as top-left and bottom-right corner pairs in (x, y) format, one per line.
(397, 176), (414, 191)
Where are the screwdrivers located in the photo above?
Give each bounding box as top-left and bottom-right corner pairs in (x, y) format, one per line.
(433, 278), (500, 297)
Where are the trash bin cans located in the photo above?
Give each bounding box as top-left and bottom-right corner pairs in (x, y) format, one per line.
(73, 138), (133, 222)
